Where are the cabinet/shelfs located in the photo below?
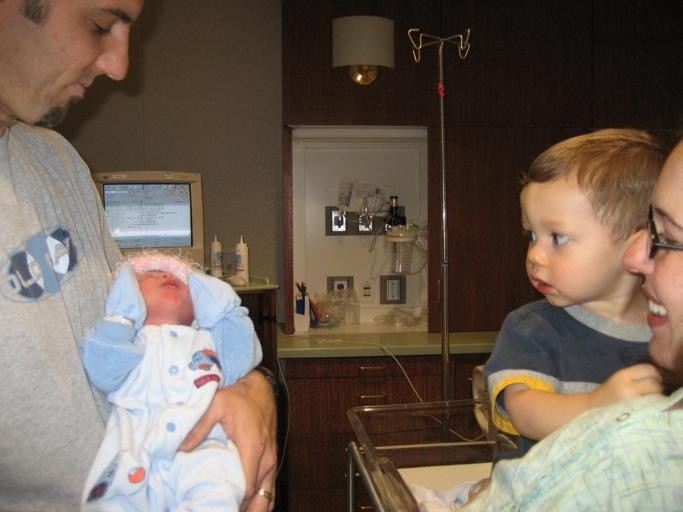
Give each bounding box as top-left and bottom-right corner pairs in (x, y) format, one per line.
(277, 353), (512, 511)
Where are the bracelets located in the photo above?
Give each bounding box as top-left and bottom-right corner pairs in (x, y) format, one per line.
(255, 364), (284, 410)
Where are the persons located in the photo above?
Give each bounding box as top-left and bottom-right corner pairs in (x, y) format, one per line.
(414, 123), (682, 512)
(0, 0), (281, 511)
(472, 128), (673, 498)
(77, 246), (264, 511)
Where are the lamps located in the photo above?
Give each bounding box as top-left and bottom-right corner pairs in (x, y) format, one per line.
(331, 16), (394, 87)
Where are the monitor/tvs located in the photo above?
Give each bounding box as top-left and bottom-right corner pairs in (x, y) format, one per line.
(91, 170), (205, 267)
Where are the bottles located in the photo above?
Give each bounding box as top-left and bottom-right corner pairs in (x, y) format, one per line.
(383, 195), (408, 233)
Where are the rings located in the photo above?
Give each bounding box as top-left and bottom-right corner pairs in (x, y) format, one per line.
(255, 487), (273, 503)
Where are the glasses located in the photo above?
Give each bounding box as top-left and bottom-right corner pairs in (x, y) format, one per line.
(648, 203), (683, 258)
